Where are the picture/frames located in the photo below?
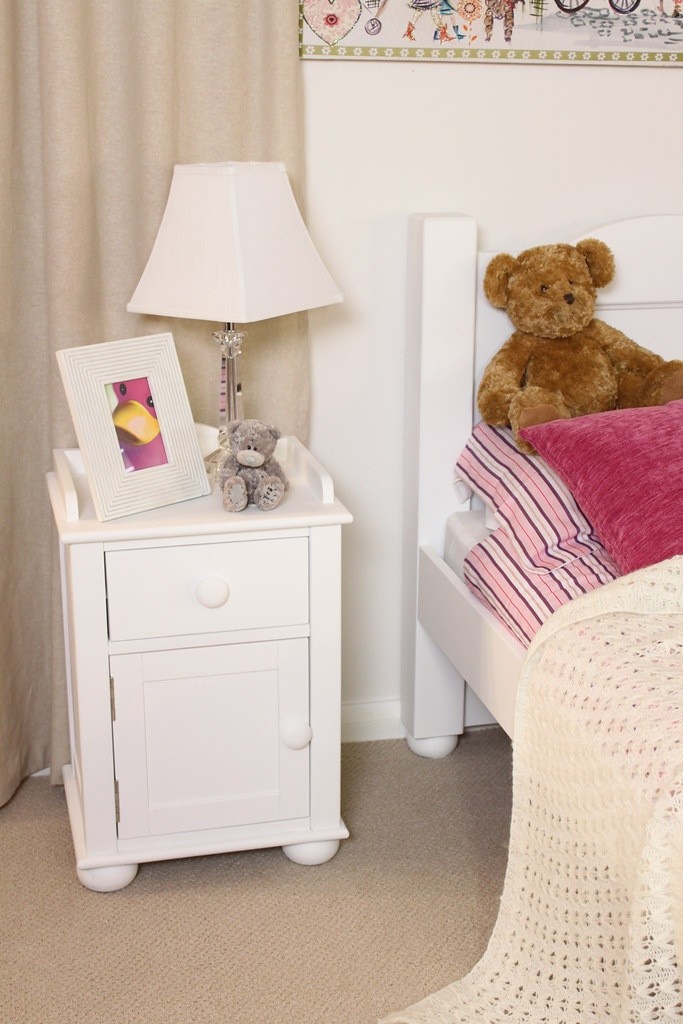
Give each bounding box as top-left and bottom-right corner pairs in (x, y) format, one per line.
(56, 331), (212, 525)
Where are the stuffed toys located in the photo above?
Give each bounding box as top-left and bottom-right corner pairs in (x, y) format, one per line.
(475, 241), (683, 455)
(214, 420), (289, 513)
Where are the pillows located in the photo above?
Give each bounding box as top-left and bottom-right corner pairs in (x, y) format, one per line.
(518, 398), (683, 579)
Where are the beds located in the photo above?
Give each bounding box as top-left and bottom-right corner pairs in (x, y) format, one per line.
(400, 209), (683, 1023)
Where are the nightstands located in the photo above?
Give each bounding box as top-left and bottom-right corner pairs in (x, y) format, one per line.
(44, 434), (355, 896)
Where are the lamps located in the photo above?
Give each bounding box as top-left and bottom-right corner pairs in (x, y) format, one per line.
(126, 158), (345, 481)
